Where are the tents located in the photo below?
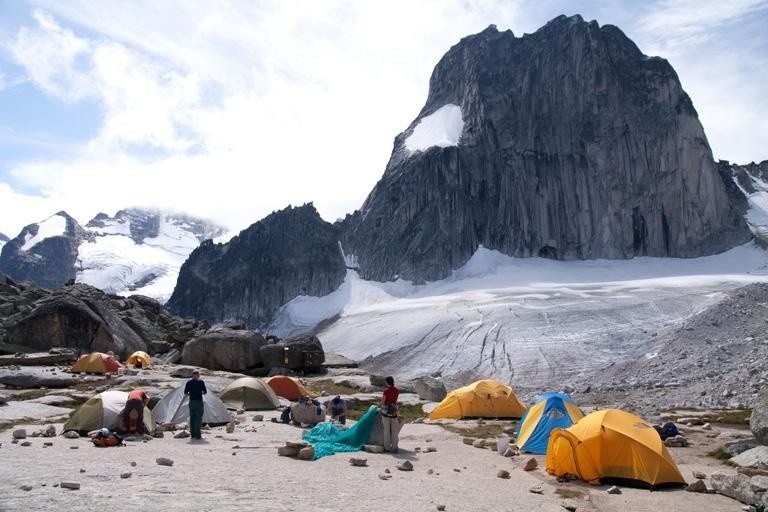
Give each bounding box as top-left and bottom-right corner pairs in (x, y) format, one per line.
(126, 350), (153, 370)
(150, 382), (233, 428)
(218, 377), (279, 410)
(514, 390), (586, 454)
(264, 374), (317, 402)
(59, 388), (158, 438)
(65, 351), (123, 374)
(544, 409), (689, 489)
(428, 378), (528, 424)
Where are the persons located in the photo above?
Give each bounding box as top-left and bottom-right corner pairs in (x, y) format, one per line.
(326, 395), (347, 424)
(379, 375), (401, 453)
(281, 407), (294, 424)
(122, 388), (151, 437)
(184, 370), (208, 439)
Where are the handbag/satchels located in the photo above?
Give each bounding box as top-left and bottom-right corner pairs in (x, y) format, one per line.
(381, 404), (397, 418)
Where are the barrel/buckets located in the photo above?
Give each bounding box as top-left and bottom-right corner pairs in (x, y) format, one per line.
(106, 373), (111, 379)
(496, 437), (510, 455)
(117, 368), (123, 375)
